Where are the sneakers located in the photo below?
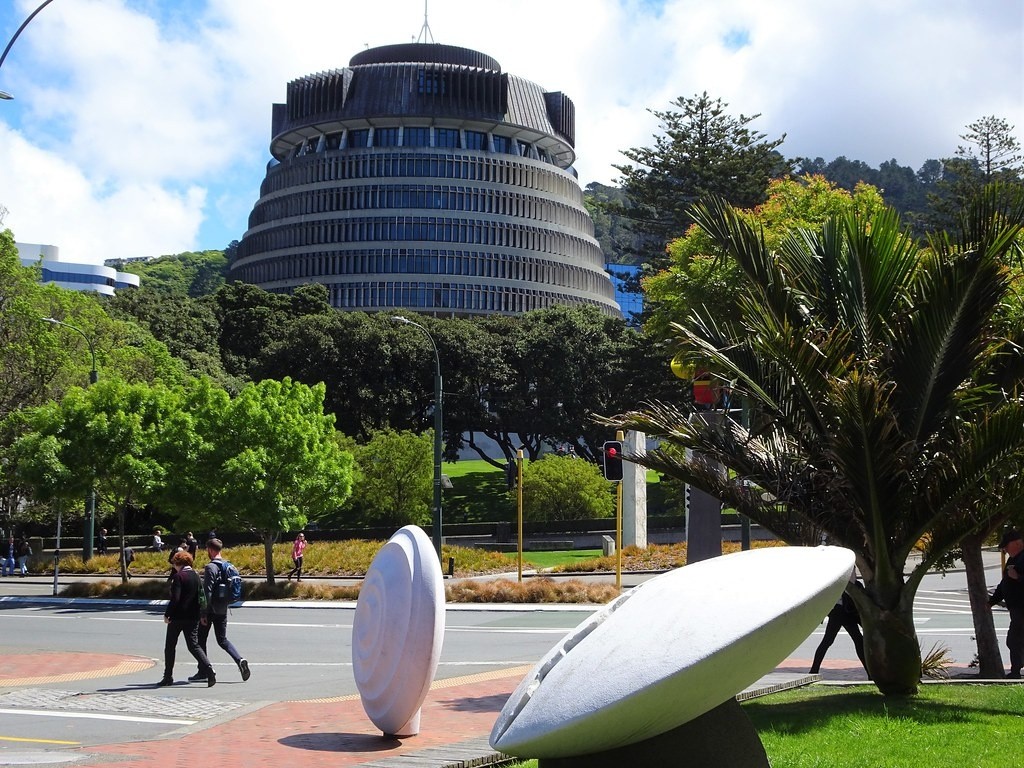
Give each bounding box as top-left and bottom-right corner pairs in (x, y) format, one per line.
(188, 671), (209, 681)
(238, 658), (250, 681)
(297, 580), (303, 582)
(288, 574), (291, 581)
(207, 668), (216, 687)
(157, 677), (173, 686)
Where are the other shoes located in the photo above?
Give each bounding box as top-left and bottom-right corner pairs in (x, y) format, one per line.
(129, 575), (132, 579)
(26, 572), (29, 577)
(20, 575), (25, 578)
(1, 574), (7, 577)
(9, 574), (14, 577)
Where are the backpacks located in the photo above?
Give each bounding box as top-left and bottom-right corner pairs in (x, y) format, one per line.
(210, 559), (242, 606)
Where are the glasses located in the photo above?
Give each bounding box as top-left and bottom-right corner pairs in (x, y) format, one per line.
(300, 536), (305, 537)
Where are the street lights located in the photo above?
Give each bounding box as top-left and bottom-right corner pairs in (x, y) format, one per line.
(41, 318), (97, 562)
(391, 316), (441, 567)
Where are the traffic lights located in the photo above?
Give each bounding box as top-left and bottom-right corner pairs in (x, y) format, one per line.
(505, 458), (518, 491)
(603, 441), (624, 481)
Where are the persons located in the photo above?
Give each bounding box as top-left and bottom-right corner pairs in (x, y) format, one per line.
(167, 538), (187, 583)
(187, 538), (251, 683)
(17, 536), (29, 577)
(97, 528), (108, 557)
(118, 540), (134, 579)
(186, 531), (198, 563)
(984, 532), (1023, 679)
(810, 542), (873, 681)
(1, 537), (16, 577)
(153, 530), (165, 553)
(208, 528), (217, 539)
(156, 550), (217, 687)
(287, 533), (307, 582)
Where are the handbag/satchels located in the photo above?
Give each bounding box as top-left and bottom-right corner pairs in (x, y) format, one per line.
(130, 555), (135, 561)
(25, 546), (33, 557)
